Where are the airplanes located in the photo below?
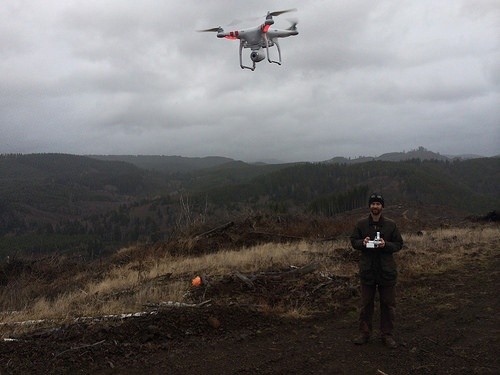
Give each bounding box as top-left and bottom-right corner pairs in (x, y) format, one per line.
(194, 8), (299, 71)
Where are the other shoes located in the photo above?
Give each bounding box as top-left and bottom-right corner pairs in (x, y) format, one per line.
(381, 335), (397, 348)
(353, 332), (370, 343)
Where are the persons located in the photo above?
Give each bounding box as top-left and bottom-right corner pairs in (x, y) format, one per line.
(349, 192), (403, 349)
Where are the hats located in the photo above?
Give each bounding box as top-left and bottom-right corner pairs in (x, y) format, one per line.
(368, 192), (385, 208)
